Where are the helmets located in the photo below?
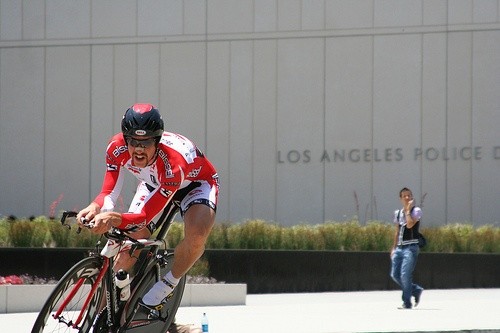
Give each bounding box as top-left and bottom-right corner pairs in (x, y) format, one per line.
(121, 104), (164, 136)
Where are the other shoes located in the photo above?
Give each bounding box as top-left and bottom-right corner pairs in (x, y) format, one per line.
(397, 303), (411, 309)
(414, 288), (423, 308)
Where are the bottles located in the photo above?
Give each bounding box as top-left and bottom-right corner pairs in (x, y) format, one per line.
(201, 313), (208, 333)
(115, 269), (130, 301)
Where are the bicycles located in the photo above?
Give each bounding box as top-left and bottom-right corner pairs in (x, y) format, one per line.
(31, 203), (187, 333)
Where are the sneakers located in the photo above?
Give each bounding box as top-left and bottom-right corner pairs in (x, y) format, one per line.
(142, 276), (178, 306)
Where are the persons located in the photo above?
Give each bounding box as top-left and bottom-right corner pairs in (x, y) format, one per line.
(389, 187), (423, 309)
(74, 103), (219, 318)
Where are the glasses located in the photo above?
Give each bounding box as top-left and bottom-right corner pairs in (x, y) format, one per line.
(125, 137), (156, 148)
